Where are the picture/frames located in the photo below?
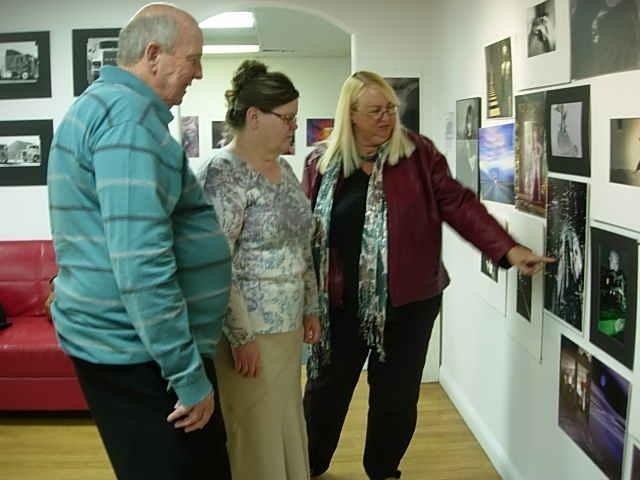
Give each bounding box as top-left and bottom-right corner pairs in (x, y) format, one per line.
(70, 28), (124, 96)
(546, 82), (591, 178)
(0, 30), (52, 100)
(586, 219), (638, 375)
(538, 173), (590, 341)
(0, 119), (55, 187)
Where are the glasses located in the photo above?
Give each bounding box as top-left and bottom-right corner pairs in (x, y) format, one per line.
(265, 111), (296, 125)
(352, 104), (399, 120)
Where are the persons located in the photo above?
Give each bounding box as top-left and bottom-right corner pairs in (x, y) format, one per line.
(302, 71), (557, 480)
(48, 2), (231, 480)
(461, 104), (475, 190)
(198, 60), (322, 479)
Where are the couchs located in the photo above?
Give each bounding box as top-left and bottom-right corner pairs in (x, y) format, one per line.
(1, 241), (89, 413)
